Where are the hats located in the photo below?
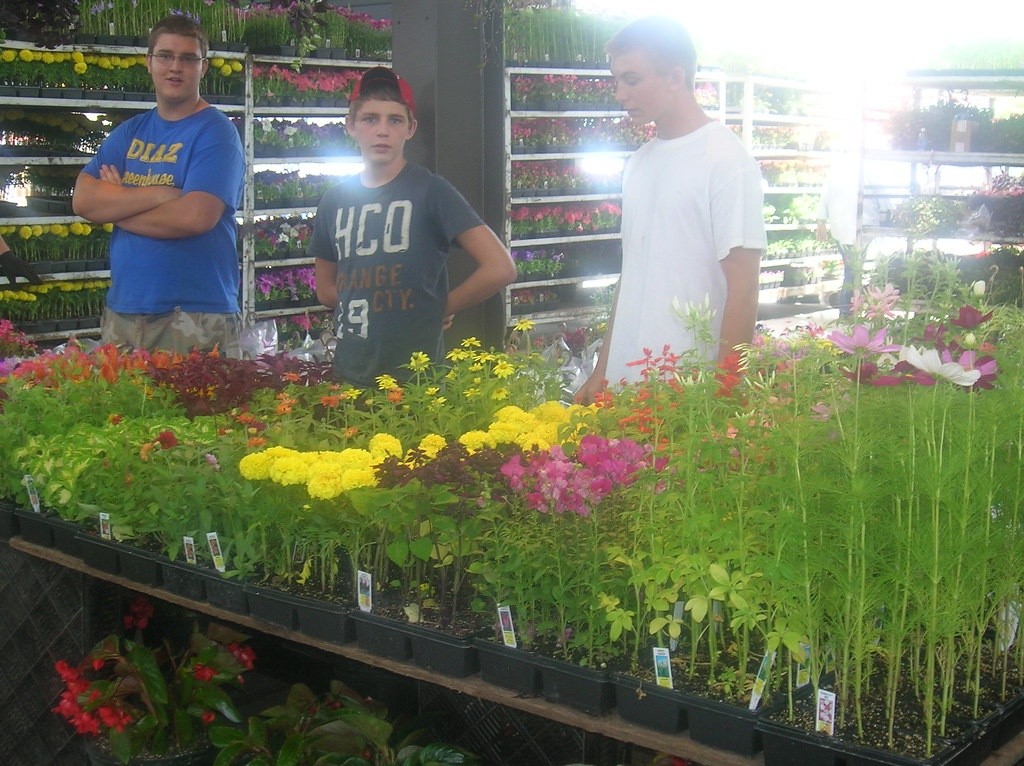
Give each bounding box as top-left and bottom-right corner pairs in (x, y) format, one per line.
(349, 66), (414, 109)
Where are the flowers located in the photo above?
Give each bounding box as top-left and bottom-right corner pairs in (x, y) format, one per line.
(1, 1), (1024, 766)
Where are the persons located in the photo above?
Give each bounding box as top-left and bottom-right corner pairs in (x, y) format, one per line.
(0, 234), (43, 292)
(305, 66), (517, 393)
(73, 15), (247, 358)
(574, 15), (768, 406)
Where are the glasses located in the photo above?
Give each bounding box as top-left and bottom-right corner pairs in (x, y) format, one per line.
(149, 53), (206, 66)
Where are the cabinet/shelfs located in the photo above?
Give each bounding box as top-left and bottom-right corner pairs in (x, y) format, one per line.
(0, 38), (394, 342)
(853, 72), (1024, 258)
(484, 64), (854, 349)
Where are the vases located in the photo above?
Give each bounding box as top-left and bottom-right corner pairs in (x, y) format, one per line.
(348, 609), (489, 679)
(74, 533), (159, 585)
(156, 554), (249, 615)
(469, 636), (617, 715)
(245, 585), (357, 646)
(609, 672), (763, 758)
(1, 502), (21, 539)
(753, 673), (1024, 766)
(14, 507), (81, 554)
